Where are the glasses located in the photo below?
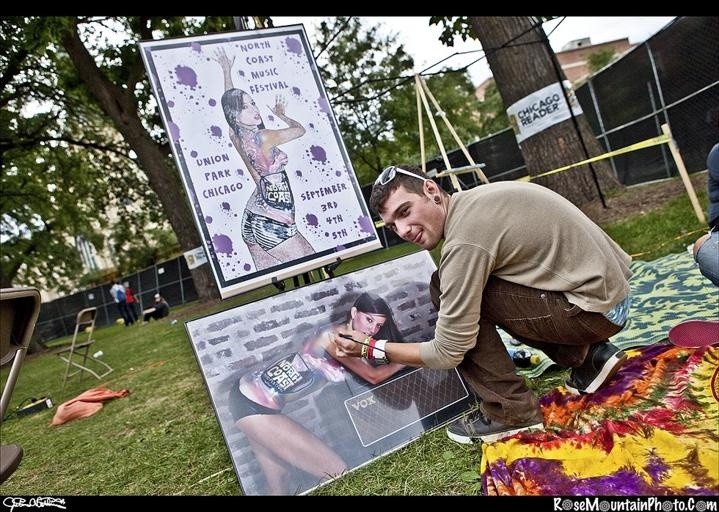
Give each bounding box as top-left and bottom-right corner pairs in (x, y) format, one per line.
(373, 165), (427, 187)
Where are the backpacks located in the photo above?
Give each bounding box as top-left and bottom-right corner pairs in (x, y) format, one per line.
(113, 286), (126, 302)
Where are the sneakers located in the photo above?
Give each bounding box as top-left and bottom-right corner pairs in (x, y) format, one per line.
(446, 410), (545, 445)
(564, 338), (628, 395)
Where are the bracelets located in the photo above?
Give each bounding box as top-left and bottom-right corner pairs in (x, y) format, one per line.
(361, 336), (372, 358)
(373, 339), (391, 366)
(368, 339), (376, 359)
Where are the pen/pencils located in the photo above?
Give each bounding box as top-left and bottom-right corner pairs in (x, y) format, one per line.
(342, 336), (391, 354)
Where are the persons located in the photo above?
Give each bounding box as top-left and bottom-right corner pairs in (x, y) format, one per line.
(229, 291), (418, 494)
(332, 162), (634, 444)
(109, 280), (135, 327)
(208, 47), (318, 271)
(141, 292), (170, 325)
(693, 143), (719, 287)
(121, 281), (138, 321)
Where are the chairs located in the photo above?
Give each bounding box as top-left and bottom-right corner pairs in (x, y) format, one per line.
(54, 303), (112, 389)
(0, 285), (43, 484)
